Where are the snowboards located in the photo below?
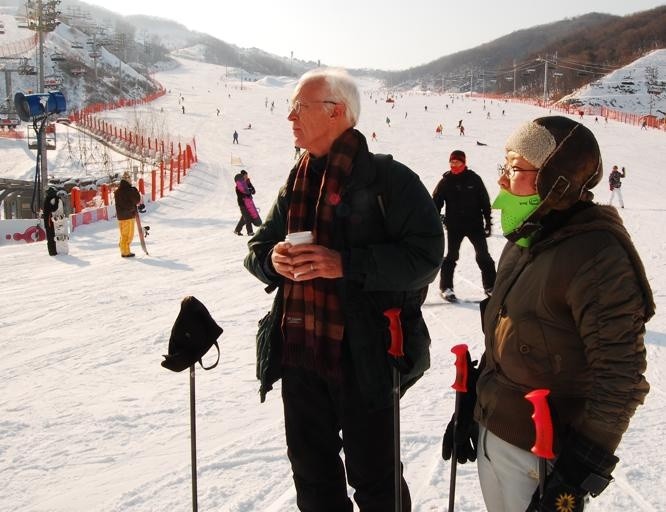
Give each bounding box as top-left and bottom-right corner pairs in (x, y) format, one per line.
(234, 173), (262, 227)
(50, 198), (70, 255)
(422, 293), (488, 306)
(137, 204), (152, 255)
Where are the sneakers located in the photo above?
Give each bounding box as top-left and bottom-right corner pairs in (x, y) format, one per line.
(233, 230), (244, 237)
(121, 252), (136, 258)
(247, 231), (256, 236)
(440, 286), (457, 302)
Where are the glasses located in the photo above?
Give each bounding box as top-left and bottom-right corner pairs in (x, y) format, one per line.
(286, 99), (339, 114)
(495, 163), (540, 177)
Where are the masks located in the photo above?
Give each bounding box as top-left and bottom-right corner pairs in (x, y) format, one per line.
(450, 164), (465, 174)
(490, 188), (540, 248)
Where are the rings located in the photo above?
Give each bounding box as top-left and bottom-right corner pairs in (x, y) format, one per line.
(309, 263), (315, 272)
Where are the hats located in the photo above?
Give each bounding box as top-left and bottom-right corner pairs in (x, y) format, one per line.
(449, 150), (466, 162)
(122, 171), (132, 178)
(241, 169), (247, 176)
(159, 296), (224, 374)
(504, 114), (604, 211)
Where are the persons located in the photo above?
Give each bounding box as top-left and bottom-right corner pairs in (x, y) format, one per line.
(183, 84), (648, 159)
(608, 166), (625, 208)
(244, 68), (443, 512)
(443, 115), (656, 512)
(115, 171), (140, 257)
(233, 170), (255, 235)
(433, 150), (496, 301)
(43, 187), (61, 256)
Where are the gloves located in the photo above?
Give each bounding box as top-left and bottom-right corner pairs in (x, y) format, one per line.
(440, 352), (486, 465)
(522, 433), (620, 512)
